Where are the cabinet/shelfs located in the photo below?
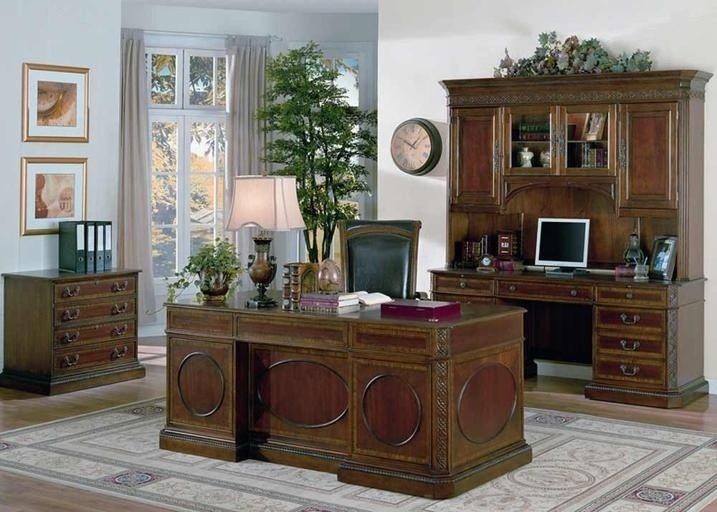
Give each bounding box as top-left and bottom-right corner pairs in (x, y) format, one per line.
(429, 69), (715, 410)
(0, 266), (146, 397)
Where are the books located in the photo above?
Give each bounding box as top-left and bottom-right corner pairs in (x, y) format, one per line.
(299, 288), (395, 316)
(462, 233), (498, 264)
(517, 113), (550, 141)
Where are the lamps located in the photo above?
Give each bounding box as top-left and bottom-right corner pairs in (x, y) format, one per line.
(226, 172), (307, 310)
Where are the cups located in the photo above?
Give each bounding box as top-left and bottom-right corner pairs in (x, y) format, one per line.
(634, 265), (649, 280)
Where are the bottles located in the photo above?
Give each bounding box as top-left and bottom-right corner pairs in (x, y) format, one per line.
(516, 147), (534, 168)
(539, 146), (550, 168)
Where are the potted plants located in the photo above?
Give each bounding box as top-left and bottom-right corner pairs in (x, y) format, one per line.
(143, 236), (245, 315)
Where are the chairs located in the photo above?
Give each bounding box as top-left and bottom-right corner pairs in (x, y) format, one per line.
(336, 217), (421, 300)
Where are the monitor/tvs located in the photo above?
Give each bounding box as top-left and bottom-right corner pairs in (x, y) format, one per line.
(534, 217), (590, 278)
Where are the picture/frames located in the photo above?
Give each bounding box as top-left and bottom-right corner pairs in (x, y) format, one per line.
(23, 63), (90, 143)
(19, 156), (89, 236)
(649, 234), (678, 281)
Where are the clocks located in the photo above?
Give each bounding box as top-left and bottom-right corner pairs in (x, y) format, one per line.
(389, 117), (442, 177)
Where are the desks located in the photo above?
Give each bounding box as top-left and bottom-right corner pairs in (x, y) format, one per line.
(159, 298), (533, 501)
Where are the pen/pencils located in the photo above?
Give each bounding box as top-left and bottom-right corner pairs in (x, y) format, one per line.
(633, 257), (648, 265)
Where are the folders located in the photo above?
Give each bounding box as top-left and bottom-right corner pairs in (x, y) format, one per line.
(59, 221), (112, 272)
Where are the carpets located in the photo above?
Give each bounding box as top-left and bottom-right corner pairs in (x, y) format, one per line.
(0, 395), (717, 512)
